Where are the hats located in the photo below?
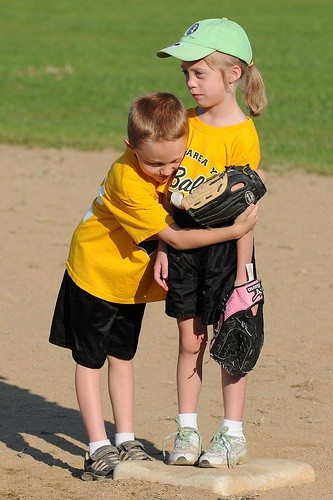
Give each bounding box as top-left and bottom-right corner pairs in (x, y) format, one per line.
(156, 17), (253, 65)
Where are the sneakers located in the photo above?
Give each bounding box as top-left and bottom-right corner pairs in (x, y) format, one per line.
(80, 445), (121, 481)
(162, 416), (201, 465)
(115, 440), (159, 462)
(198, 426), (249, 469)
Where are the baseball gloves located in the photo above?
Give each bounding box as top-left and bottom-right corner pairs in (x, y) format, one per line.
(209, 258), (265, 379)
(169, 164), (267, 230)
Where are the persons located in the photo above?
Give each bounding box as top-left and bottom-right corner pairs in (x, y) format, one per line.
(154, 17), (267, 468)
(47, 93), (260, 482)
